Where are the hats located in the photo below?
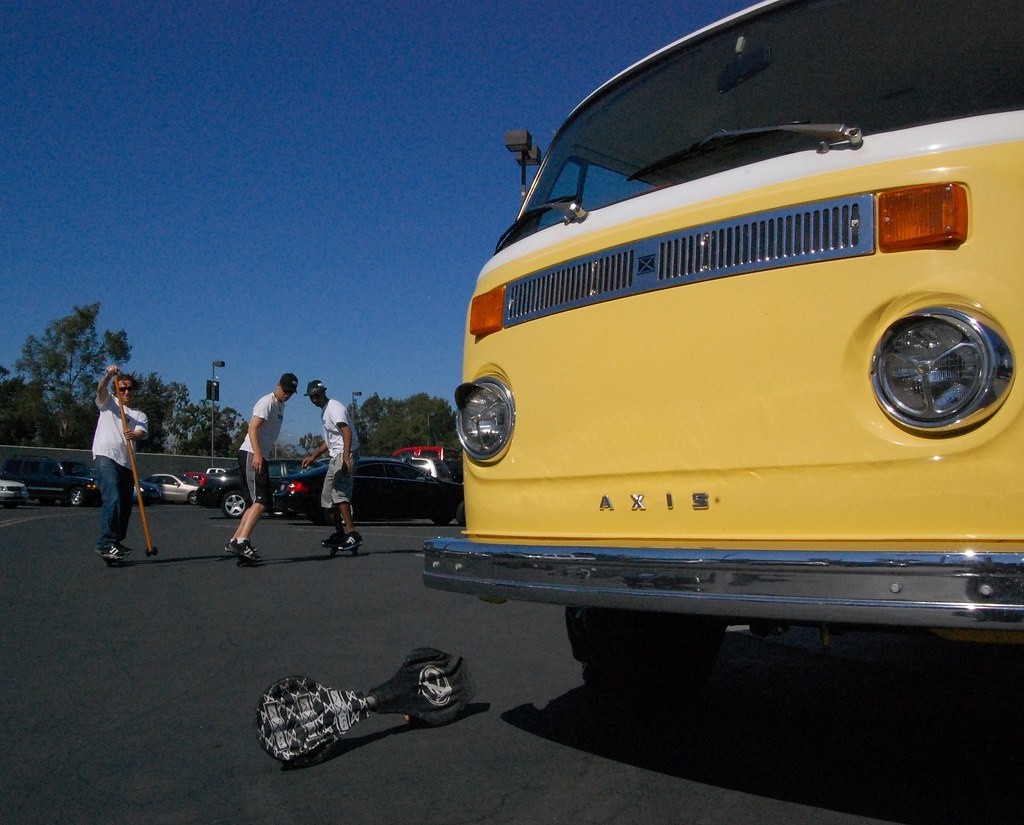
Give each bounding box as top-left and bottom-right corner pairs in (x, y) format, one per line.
(304, 380), (327, 396)
(280, 373), (298, 393)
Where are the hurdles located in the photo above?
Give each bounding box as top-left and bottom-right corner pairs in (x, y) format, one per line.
(324, 537), (362, 556)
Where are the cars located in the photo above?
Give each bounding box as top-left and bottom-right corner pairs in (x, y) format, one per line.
(393, 446), (464, 482)
(201, 456), (331, 520)
(0, 479), (29, 509)
(270, 454), (466, 535)
(142, 473), (202, 505)
(206, 467), (233, 476)
(179, 471), (209, 488)
(90, 467), (161, 504)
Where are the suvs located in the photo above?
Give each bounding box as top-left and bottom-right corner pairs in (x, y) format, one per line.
(0, 453), (105, 508)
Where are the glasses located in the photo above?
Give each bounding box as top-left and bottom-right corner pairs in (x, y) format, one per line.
(119, 386), (134, 392)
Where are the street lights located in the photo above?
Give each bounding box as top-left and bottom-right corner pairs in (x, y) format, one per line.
(350, 391), (362, 426)
(211, 360), (227, 467)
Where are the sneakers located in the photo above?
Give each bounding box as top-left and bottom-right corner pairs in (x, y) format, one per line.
(225, 539), (258, 553)
(337, 532), (363, 550)
(231, 539), (261, 561)
(321, 532), (346, 547)
(115, 542), (132, 555)
(95, 545), (128, 560)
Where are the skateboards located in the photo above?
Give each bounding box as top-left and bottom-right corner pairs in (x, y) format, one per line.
(223, 548), (260, 567)
(253, 644), (476, 769)
(102, 555), (126, 567)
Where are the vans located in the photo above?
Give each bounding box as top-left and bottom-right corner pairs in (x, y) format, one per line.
(422, 0), (1023, 649)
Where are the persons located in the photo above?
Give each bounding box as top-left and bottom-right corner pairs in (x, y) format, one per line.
(92, 365), (148, 559)
(304, 380), (363, 550)
(225, 373), (299, 560)
